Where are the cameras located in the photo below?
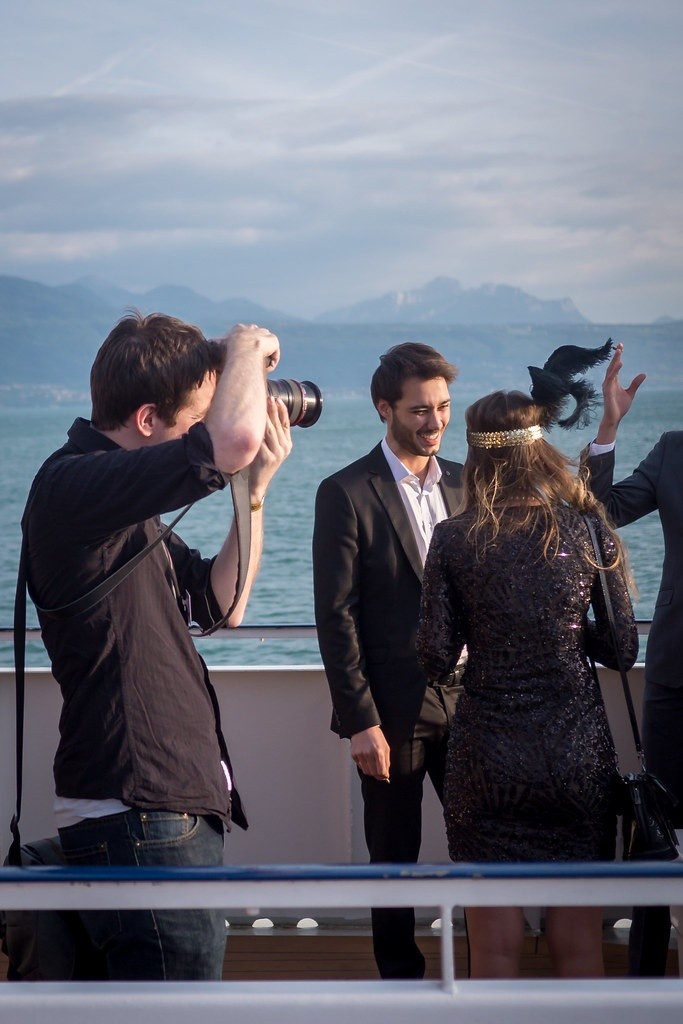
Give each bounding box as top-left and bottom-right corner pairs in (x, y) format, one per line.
(264, 355), (323, 428)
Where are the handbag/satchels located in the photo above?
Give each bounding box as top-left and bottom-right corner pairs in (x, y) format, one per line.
(0, 835), (77, 983)
(626, 771), (680, 861)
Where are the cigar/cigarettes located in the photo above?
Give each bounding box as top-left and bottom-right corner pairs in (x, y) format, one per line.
(386, 778), (390, 783)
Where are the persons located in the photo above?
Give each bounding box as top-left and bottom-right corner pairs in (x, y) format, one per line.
(414, 386), (640, 981)
(16, 311), (295, 981)
(311, 341), (478, 981)
(580, 340), (683, 978)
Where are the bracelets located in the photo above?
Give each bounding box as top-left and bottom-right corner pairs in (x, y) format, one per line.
(250, 496), (265, 511)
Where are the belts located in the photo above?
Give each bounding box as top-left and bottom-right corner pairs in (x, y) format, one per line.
(426, 661), (467, 686)
